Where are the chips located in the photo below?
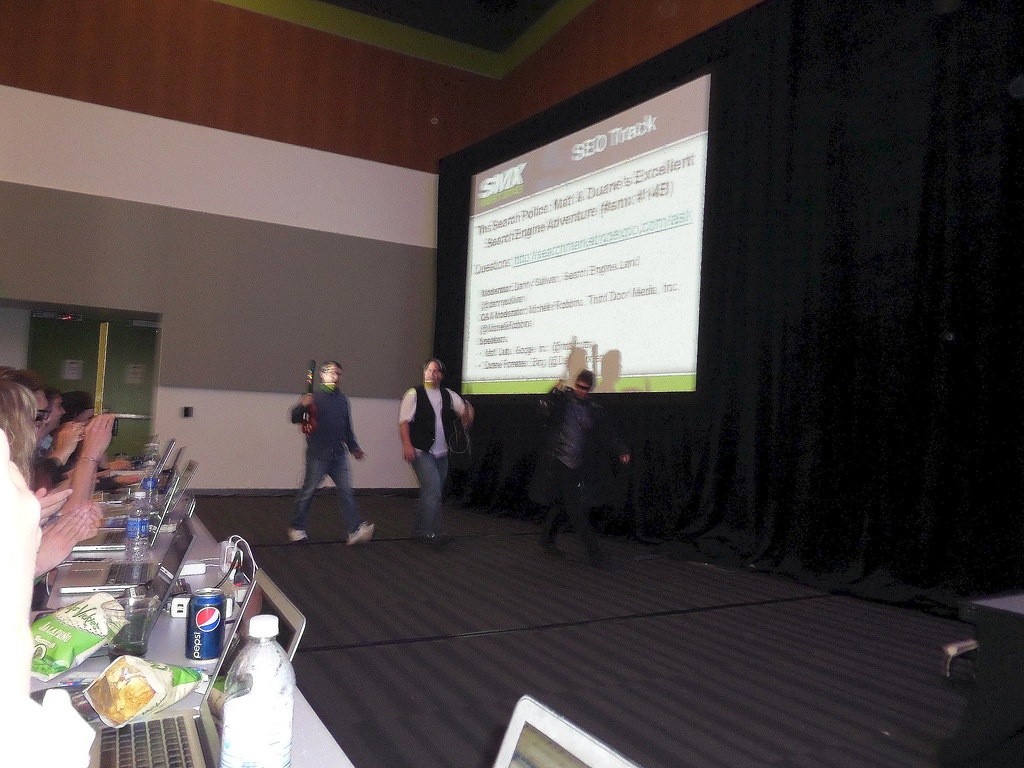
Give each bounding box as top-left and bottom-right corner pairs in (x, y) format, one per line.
(83, 656), (209, 728)
(30, 591), (131, 683)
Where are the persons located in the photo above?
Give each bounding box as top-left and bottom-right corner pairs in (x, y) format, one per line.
(397, 358), (475, 552)
(287, 361), (377, 548)
(536, 369), (631, 574)
(1, 366), (146, 768)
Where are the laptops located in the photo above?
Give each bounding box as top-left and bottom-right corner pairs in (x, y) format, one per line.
(86, 569), (306, 768)
(491, 693), (640, 768)
(30, 512), (197, 660)
(60, 489), (197, 596)
(95, 434), (187, 504)
(72, 459), (199, 553)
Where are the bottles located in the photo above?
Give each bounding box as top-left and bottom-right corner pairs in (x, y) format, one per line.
(143, 471), (159, 506)
(125, 491), (150, 562)
(219, 612), (299, 767)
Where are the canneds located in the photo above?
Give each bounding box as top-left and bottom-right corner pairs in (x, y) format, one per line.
(183, 587), (224, 660)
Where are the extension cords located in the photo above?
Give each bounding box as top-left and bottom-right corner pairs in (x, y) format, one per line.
(216, 570), (251, 603)
(171, 594), (235, 618)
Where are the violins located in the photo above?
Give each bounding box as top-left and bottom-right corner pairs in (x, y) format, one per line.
(299, 360), (321, 436)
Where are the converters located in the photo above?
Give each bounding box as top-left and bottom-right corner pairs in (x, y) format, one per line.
(180, 560), (206, 576)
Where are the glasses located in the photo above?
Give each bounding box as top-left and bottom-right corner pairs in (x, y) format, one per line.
(37, 408), (53, 420)
(575, 384), (591, 391)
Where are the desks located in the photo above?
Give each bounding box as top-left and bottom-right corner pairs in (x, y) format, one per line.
(29, 458), (356, 768)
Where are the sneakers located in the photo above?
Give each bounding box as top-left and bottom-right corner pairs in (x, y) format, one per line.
(287, 527), (308, 543)
(346, 526), (374, 547)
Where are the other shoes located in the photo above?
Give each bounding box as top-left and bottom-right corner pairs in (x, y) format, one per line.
(576, 549), (609, 567)
(414, 536), (448, 552)
(536, 542), (566, 565)
